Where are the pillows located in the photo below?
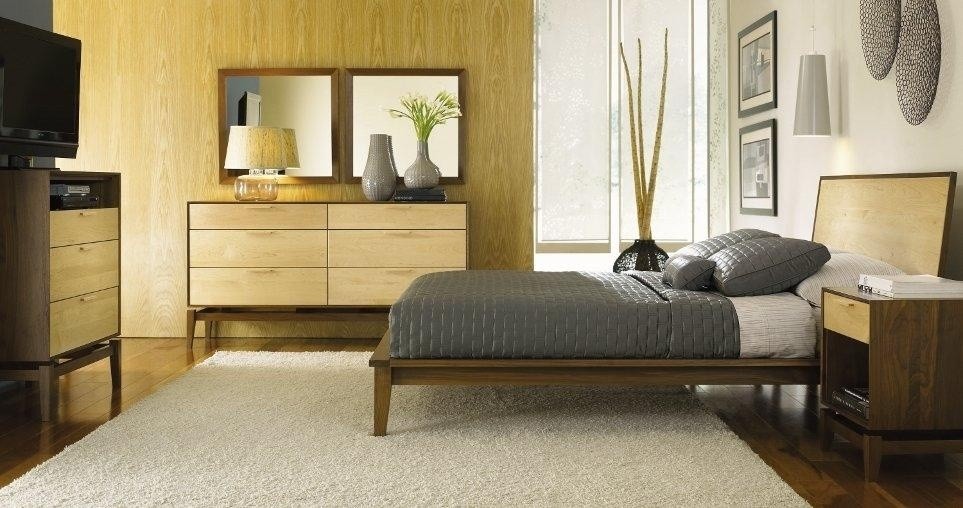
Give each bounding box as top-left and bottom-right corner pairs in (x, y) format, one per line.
(708, 237), (832, 297)
(661, 255), (716, 290)
(674, 229), (781, 259)
(794, 253), (906, 307)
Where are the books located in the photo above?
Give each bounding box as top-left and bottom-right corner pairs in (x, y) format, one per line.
(832, 388), (869, 420)
(860, 271), (963, 294)
(858, 284), (962, 300)
(842, 383), (870, 403)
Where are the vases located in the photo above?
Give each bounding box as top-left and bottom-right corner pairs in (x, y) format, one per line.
(361, 134), (397, 201)
(404, 141), (440, 189)
(614, 240), (669, 273)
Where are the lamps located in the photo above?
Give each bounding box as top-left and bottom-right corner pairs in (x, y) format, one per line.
(793, 0), (832, 136)
(223, 126), (286, 201)
(266, 129), (301, 175)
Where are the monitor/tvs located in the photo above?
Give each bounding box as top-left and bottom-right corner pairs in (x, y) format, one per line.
(238, 92), (261, 128)
(0, 13), (82, 172)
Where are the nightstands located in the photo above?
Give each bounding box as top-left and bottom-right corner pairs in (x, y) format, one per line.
(820, 286), (963, 482)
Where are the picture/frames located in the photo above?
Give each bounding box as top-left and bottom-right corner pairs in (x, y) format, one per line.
(739, 118), (778, 217)
(738, 10), (777, 119)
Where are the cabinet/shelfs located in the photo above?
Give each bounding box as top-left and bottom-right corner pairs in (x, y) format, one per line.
(0, 170), (120, 423)
(185, 201), (469, 350)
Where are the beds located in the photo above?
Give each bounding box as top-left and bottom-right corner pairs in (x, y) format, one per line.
(369, 171), (957, 436)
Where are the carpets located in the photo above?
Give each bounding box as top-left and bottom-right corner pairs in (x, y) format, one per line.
(0, 350), (814, 508)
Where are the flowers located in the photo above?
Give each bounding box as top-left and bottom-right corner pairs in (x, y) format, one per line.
(385, 89), (462, 142)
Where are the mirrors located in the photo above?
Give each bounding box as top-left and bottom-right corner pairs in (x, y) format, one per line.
(218, 68), (340, 184)
(345, 68), (467, 185)
